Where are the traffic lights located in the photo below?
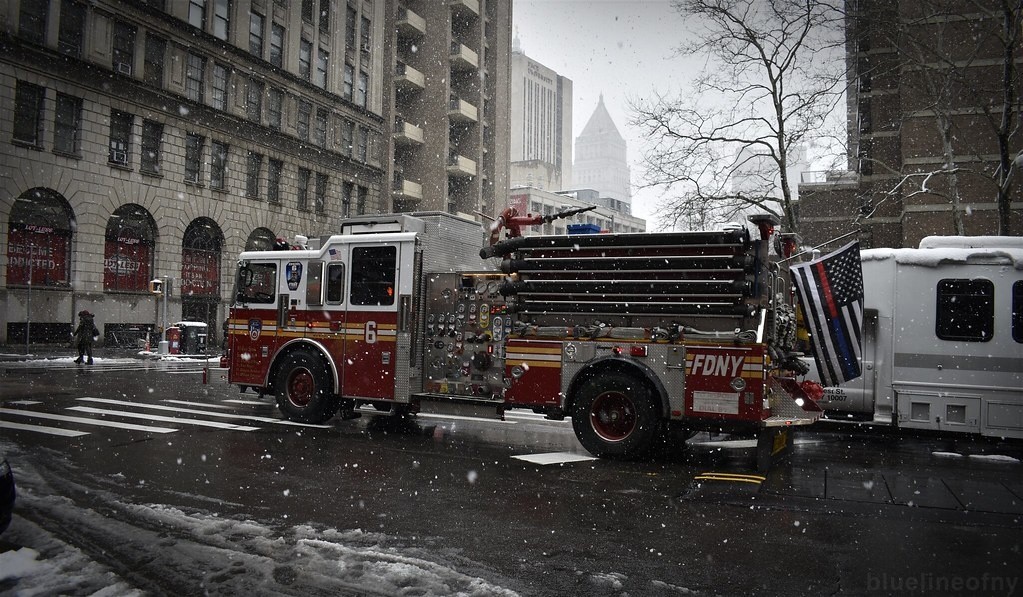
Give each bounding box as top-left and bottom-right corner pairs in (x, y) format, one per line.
(150, 279), (162, 292)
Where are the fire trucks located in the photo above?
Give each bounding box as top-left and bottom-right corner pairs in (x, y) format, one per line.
(219, 199), (827, 466)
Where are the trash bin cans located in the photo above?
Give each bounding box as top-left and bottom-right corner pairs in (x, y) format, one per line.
(166, 327), (182, 354)
(173, 322), (209, 355)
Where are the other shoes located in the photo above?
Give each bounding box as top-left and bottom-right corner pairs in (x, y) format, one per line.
(74, 359), (81, 364)
(85, 361), (93, 364)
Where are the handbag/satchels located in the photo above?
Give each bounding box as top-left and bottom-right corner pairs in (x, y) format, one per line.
(92, 328), (100, 336)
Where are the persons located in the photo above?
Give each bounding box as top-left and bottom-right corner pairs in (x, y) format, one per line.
(72, 310), (99, 365)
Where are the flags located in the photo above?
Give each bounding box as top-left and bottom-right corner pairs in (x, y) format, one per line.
(792, 238), (866, 388)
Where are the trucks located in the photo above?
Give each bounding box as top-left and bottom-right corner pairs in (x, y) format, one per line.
(781, 235), (1023, 456)
